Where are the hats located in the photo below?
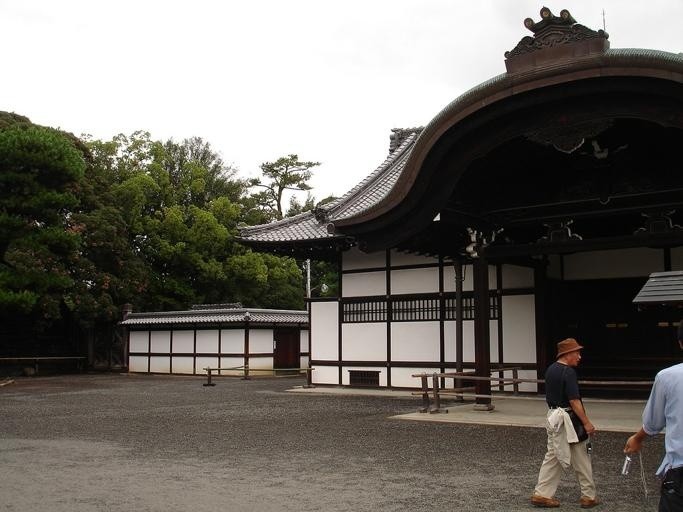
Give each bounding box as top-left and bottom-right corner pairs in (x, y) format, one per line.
(556, 337), (584, 359)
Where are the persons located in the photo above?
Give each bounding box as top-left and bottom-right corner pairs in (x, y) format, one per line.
(619, 360), (682, 512)
(531, 336), (600, 509)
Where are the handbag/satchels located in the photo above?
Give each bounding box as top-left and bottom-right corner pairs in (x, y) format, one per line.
(565, 410), (589, 443)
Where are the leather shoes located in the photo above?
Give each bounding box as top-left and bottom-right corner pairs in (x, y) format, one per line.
(550, 404), (568, 409)
(580, 498), (601, 508)
(531, 495), (560, 508)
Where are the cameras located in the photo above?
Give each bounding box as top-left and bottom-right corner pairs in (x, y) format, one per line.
(621, 456), (634, 475)
(587, 442), (592, 454)
